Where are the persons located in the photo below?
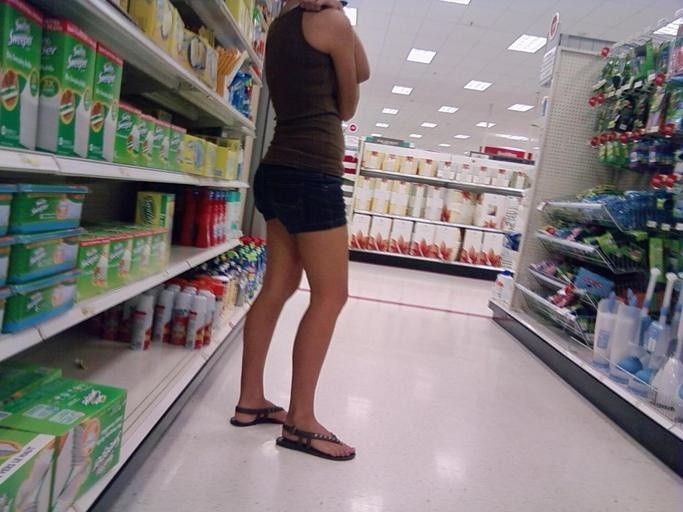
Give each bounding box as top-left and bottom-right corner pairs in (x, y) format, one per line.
(227, 0), (372, 462)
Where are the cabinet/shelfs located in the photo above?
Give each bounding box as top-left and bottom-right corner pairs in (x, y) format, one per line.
(342, 138), (535, 273)
(0, 0), (263, 512)
(516, 202), (646, 350)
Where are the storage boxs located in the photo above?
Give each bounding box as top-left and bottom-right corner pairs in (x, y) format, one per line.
(0, 363), (126, 512)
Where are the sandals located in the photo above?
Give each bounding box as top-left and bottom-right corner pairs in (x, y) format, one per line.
(231, 406), (284, 427)
(276, 424), (356, 461)
